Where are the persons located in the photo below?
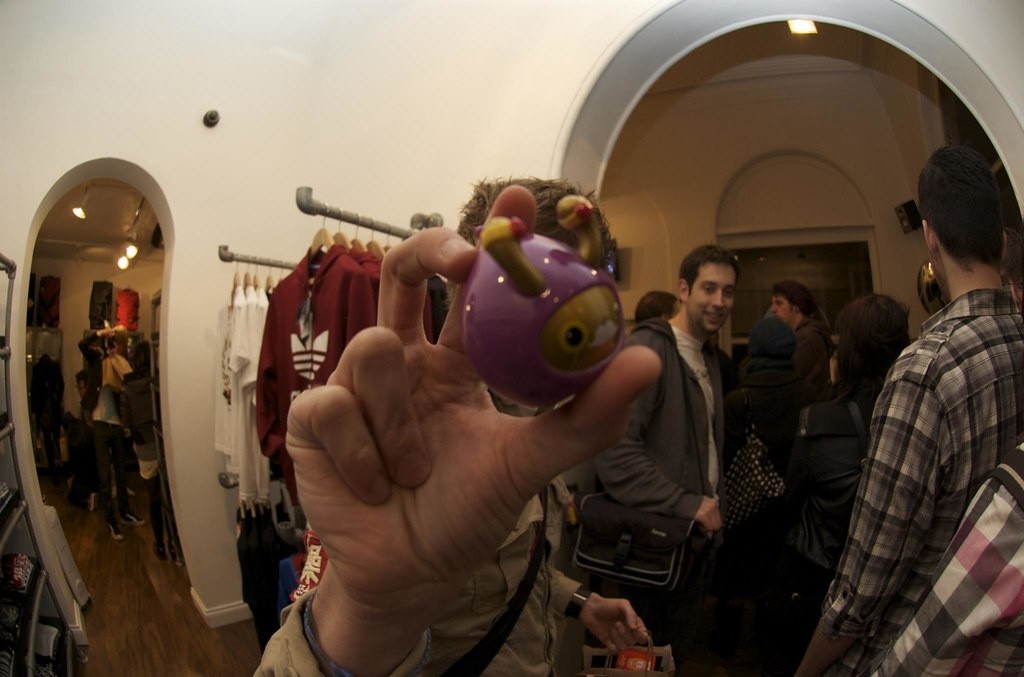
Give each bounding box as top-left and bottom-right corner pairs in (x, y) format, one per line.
(29, 145), (1024, 677)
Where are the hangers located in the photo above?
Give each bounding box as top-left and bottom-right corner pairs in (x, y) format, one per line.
(309, 203), (391, 262)
(230, 254), (284, 304)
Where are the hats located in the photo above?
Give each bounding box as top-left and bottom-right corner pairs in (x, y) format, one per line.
(749, 318), (797, 359)
(772, 281), (818, 317)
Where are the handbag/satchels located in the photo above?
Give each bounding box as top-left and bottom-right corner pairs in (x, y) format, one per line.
(109, 348), (133, 382)
(102, 356), (122, 393)
(575, 491), (695, 595)
(722, 387), (786, 531)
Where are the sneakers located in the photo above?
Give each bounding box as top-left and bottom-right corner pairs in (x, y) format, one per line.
(121, 512), (145, 526)
(106, 518), (125, 540)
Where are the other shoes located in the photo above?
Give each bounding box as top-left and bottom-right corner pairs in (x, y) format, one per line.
(87, 492), (99, 511)
(153, 542), (168, 561)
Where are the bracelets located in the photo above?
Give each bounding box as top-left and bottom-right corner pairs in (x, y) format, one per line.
(564, 586), (593, 620)
(303, 591), (433, 677)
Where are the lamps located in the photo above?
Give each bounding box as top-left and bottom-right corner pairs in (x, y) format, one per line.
(72, 185), (91, 220)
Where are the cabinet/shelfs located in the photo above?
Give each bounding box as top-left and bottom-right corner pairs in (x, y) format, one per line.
(150, 289), (185, 566)
(27, 271), (63, 450)
(0, 254), (88, 677)
